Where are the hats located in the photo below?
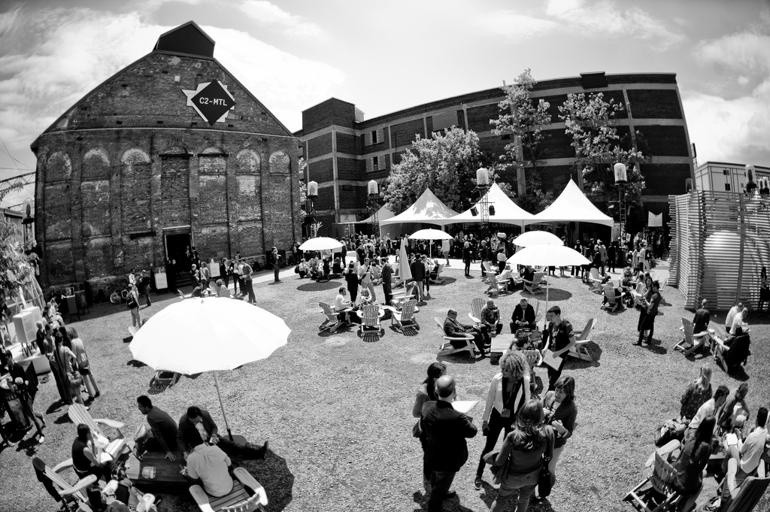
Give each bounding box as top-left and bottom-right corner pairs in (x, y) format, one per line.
(699, 367), (712, 387)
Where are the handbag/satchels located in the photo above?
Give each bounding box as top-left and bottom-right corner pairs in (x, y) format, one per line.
(66, 370), (84, 387)
(538, 469), (551, 498)
(654, 418), (683, 446)
(358, 279), (362, 285)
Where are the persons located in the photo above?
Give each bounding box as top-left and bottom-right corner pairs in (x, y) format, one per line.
(1, 296), (269, 512)
(411, 297), (770, 512)
(268, 230), (673, 346)
(124, 255), (259, 329)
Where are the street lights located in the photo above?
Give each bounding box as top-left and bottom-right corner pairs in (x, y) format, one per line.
(473, 162), (492, 235)
(367, 179), (380, 237)
(613, 161), (628, 245)
(303, 180), (319, 238)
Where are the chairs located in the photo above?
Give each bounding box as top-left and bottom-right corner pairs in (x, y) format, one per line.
(709, 333), (751, 373)
(673, 317), (709, 358)
(622, 439), (687, 512)
(67, 403), (131, 459)
(176, 288), (193, 299)
(32, 456), (99, 511)
(706, 475), (770, 512)
(189, 467), (269, 512)
